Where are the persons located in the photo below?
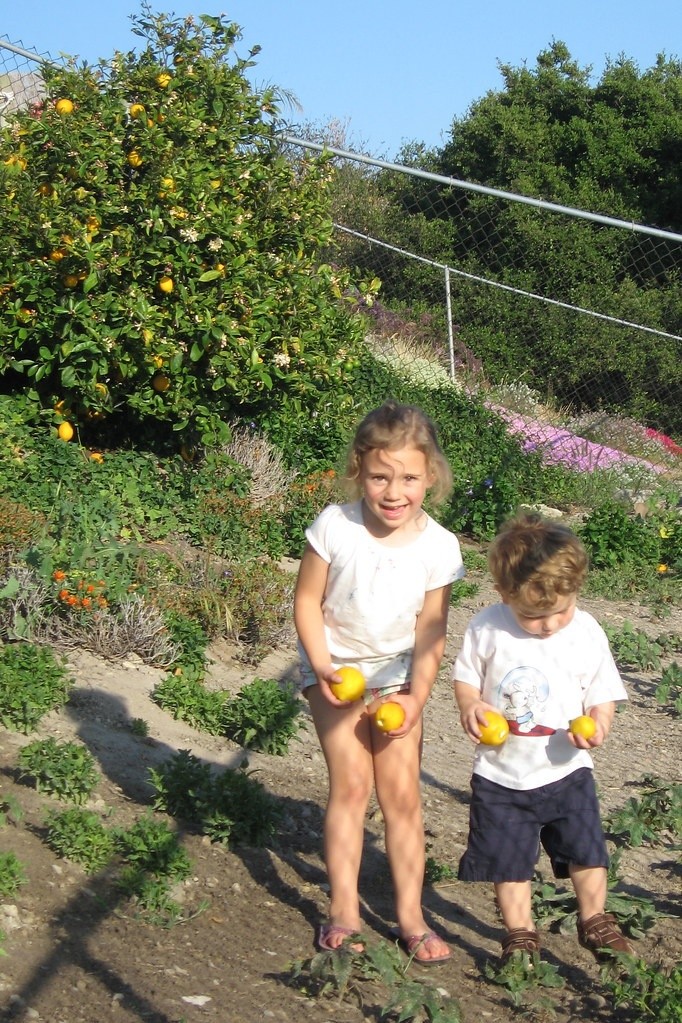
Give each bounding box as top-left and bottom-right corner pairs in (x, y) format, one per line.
(292, 401), (465, 965)
(452, 510), (638, 986)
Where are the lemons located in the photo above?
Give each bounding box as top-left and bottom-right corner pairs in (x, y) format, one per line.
(329, 666), (366, 702)
(16, 74), (361, 463)
(476, 711), (509, 746)
(569, 715), (596, 740)
(374, 701), (404, 731)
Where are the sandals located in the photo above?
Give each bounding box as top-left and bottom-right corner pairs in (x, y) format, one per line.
(576, 913), (636, 965)
(499, 927), (540, 969)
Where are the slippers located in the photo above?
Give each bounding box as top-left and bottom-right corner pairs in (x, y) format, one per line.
(389, 926), (452, 966)
(319, 923), (364, 956)
